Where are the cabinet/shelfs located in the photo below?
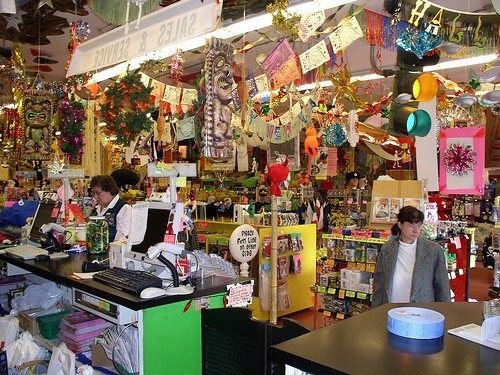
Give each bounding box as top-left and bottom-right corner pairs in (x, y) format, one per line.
(193, 220), (316, 321)
(310, 233), (388, 318)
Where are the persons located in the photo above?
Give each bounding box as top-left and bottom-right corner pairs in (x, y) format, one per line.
(372, 206), (451, 308)
(90, 174), (133, 244)
(347, 171), (367, 190)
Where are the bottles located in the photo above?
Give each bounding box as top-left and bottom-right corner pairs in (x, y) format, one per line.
(87, 216), (109, 255)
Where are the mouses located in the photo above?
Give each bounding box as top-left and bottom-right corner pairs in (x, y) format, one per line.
(140, 287), (165, 298)
(34, 254), (50, 261)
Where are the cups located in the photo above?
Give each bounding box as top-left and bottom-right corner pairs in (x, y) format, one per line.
(482, 300), (500, 321)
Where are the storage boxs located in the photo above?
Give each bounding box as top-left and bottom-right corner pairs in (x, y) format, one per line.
(36, 309), (79, 341)
(17, 308), (42, 334)
(89, 344), (115, 368)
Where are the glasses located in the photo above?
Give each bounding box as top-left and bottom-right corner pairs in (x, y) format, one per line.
(402, 222), (422, 230)
(91, 190), (104, 196)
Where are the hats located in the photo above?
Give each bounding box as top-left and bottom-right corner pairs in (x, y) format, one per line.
(345, 171), (360, 182)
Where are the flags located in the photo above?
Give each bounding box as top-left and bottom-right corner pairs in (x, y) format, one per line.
(297, 196), (329, 233)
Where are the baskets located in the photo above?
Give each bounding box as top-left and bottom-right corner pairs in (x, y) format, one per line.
(36, 309), (79, 340)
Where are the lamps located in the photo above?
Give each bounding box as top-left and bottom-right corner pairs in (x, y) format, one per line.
(74, 83), (98, 100)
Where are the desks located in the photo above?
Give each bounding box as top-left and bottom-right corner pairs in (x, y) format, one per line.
(0, 247), (257, 375)
(271, 302), (500, 375)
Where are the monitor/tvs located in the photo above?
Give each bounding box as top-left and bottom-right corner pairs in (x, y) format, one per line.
(125, 201), (183, 279)
(27, 200), (62, 248)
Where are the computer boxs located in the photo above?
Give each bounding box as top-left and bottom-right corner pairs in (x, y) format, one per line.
(108, 240), (152, 271)
(21, 226), (42, 248)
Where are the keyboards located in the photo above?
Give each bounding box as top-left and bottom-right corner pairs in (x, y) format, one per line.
(4, 245), (49, 260)
(93, 266), (162, 295)
